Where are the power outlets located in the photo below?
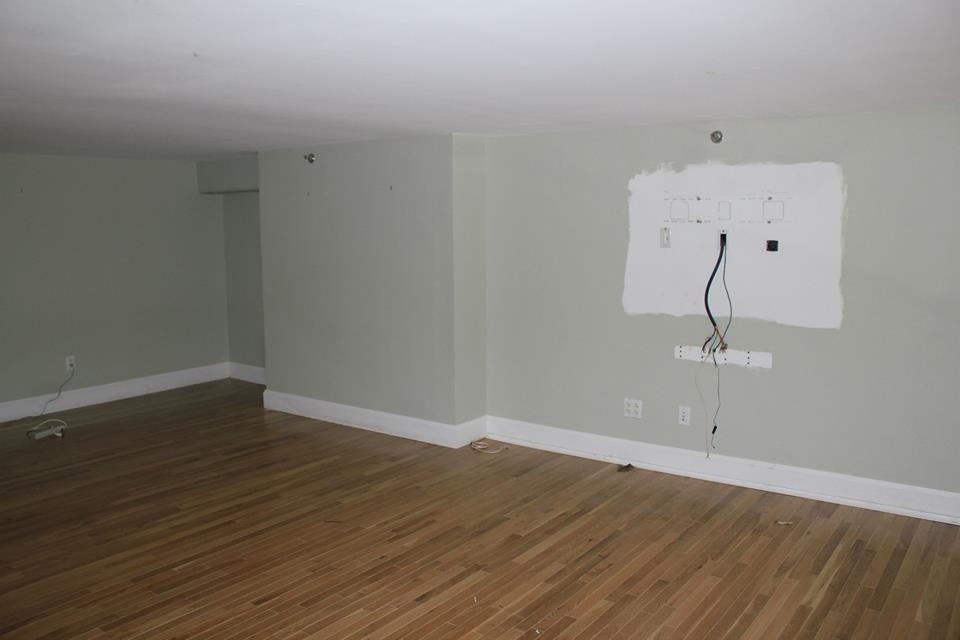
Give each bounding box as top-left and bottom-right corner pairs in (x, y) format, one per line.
(64, 354), (77, 371)
(678, 406), (692, 426)
(623, 398), (642, 418)
